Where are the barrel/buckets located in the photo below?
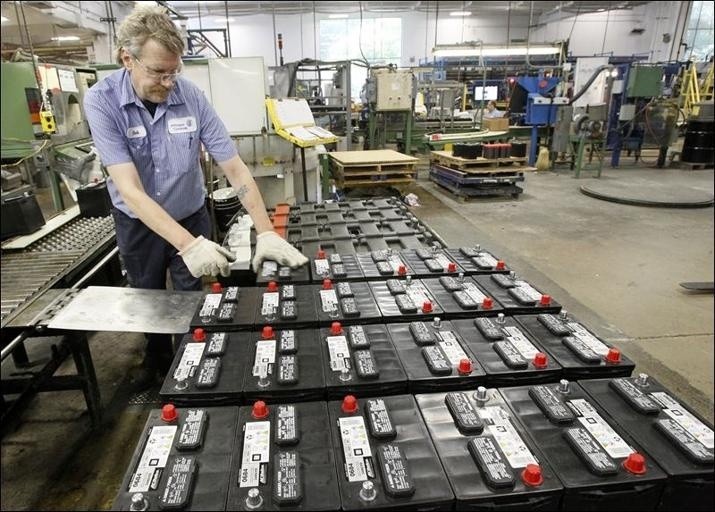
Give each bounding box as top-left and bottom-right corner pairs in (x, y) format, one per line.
(209, 187), (242, 233)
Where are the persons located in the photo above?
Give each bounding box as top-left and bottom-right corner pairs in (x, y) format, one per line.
(82, 1), (308, 378)
(484, 100), (504, 117)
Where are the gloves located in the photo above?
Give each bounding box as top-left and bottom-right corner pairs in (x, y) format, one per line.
(176, 233), (237, 279)
(251, 230), (310, 274)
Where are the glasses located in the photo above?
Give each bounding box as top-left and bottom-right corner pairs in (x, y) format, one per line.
(130, 56), (185, 82)
